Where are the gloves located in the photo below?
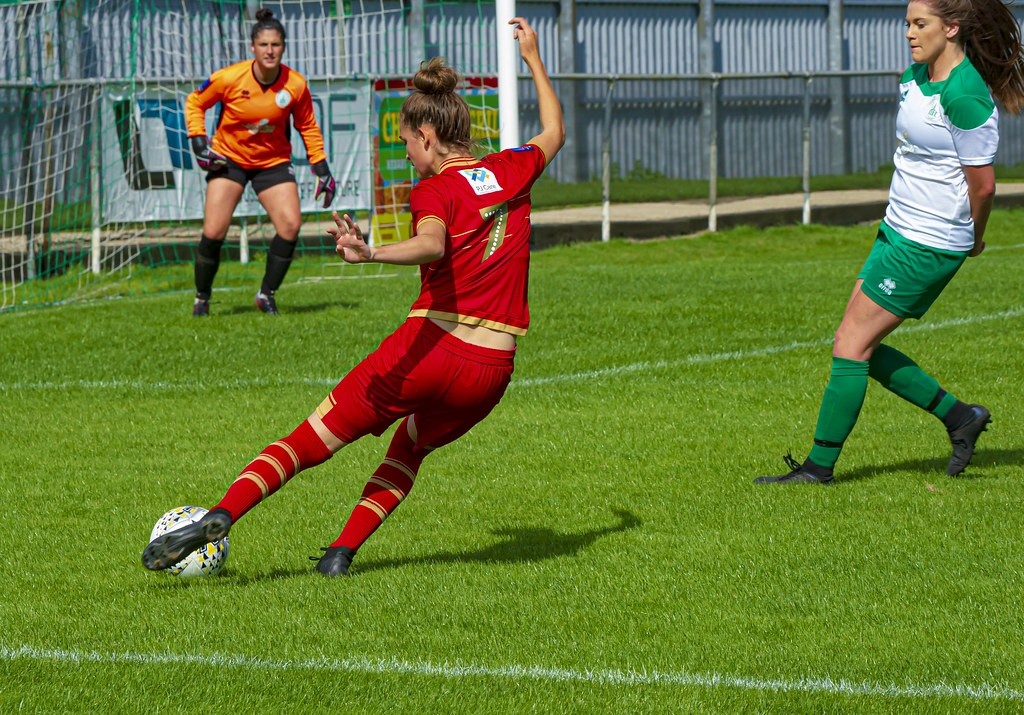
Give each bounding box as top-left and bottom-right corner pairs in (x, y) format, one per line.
(310, 159), (336, 208)
(192, 136), (227, 171)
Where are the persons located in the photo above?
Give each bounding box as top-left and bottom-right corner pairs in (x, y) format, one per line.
(139, 17), (565, 578)
(185, 8), (336, 315)
(753, 1), (1024, 486)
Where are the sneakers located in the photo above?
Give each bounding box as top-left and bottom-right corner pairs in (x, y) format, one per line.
(141, 509), (231, 571)
(309, 547), (356, 581)
(945, 404), (993, 477)
(193, 292), (209, 318)
(754, 447), (836, 487)
(256, 288), (280, 317)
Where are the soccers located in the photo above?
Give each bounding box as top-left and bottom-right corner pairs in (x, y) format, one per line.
(148, 506), (230, 579)
